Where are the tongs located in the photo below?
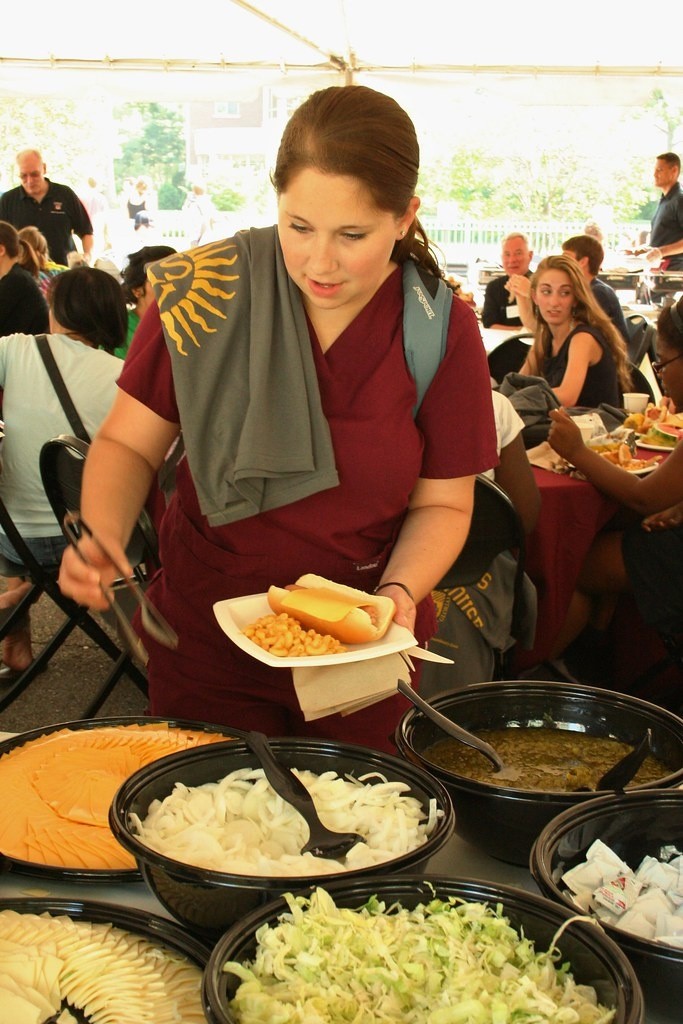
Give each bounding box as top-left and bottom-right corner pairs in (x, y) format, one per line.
(64, 510), (178, 665)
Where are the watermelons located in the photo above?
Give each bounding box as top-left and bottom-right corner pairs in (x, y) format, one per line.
(651, 423), (683, 442)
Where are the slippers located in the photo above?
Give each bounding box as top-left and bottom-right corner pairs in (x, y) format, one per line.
(0, 658), (48, 688)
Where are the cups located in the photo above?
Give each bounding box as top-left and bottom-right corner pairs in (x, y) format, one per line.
(623, 393), (650, 415)
(572, 416), (593, 442)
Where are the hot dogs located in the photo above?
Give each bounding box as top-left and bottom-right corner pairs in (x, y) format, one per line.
(642, 402), (668, 426)
(267, 574), (398, 644)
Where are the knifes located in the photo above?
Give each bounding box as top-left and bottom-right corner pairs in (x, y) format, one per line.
(401, 644), (455, 664)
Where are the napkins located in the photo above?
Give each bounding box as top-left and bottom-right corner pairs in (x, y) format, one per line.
(525, 441), (568, 475)
(290, 651), (412, 723)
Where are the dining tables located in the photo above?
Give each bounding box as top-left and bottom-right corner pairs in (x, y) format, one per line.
(0, 732), (543, 927)
(508, 446), (683, 699)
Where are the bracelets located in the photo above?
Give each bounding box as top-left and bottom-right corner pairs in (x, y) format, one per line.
(374, 582), (414, 599)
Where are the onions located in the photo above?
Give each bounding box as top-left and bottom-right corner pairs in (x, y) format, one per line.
(128, 768), (444, 877)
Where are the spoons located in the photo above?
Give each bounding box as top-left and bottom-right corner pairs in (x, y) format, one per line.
(245, 731), (366, 862)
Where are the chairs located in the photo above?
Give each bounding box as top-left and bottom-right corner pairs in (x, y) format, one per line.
(431, 471), (529, 681)
(39, 432), (160, 648)
(648, 327), (664, 396)
(487, 332), (534, 385)
(0, 498), (150, 720)
(625, 314), (652, 369)
(628, 362), (656, 406)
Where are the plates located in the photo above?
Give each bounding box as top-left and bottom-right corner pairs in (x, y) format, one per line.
(599, 453), (661, 474)
(616, 424), (677, 451)
(0, 715), (251, 883)
(0, 895), (218, 1024)
(213, 592), (419, 668)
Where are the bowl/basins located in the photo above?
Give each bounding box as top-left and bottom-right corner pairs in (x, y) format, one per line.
(201, 872), (646, 1023)
(395, 679), (683, 868)
(109, 737), (455, 944)
(529, 789), (683, 984)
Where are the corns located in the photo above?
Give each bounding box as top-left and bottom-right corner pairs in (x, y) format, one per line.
(624, 413), (645, 432)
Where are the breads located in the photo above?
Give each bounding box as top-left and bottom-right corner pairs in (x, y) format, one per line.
(599, 444), (632, 466)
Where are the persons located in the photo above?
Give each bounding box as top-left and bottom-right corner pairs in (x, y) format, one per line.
(52, 87), (495, 749)
(427, 223), (683, 715)
(0, 147), (222, 688)
(626, 151), (683, 305)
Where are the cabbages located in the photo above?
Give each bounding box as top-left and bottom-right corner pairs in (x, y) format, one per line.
(224, 887), (617, 1024)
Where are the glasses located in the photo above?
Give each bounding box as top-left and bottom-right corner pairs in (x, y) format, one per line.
(651, 353), (683, 373)
